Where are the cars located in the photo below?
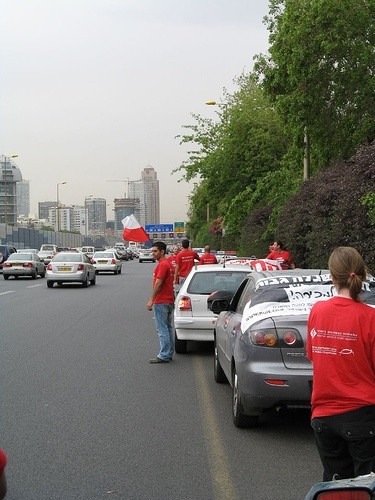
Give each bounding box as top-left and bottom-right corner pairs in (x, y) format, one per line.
(45, 253), (96, 287)
(173, 264), (252, 353)
(90, 251), (122, 275)
(37, 251), (54, 264)
(192, 247), (293, 272)
(139, 249), (156, 263)
(15, 242), (139, 264)
(2, 253), (46, 279)
(212, 268), (375, 429)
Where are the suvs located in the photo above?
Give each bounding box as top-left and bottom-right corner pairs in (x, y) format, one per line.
(0, 246), (17, 275)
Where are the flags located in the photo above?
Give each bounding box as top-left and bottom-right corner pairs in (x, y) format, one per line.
(121, 213), (149, 242)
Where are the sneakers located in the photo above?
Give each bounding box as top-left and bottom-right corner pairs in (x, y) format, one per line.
(150, 356), (169, 363)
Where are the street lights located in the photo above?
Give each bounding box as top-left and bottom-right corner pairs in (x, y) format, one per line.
(205, 101), (228, 128)
(84, 194), (93, 236)
(56, 181), (67, 231)
(4, 154), (19, 245)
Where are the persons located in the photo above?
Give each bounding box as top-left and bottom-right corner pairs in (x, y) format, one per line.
(266, 240), (296, 270)
(306, 246), (375, 482)
(167, 245), (181, 284)
(174, 240), (199, 289)
(198, 245), (218, 264)
(146, 242), (175, 364)
